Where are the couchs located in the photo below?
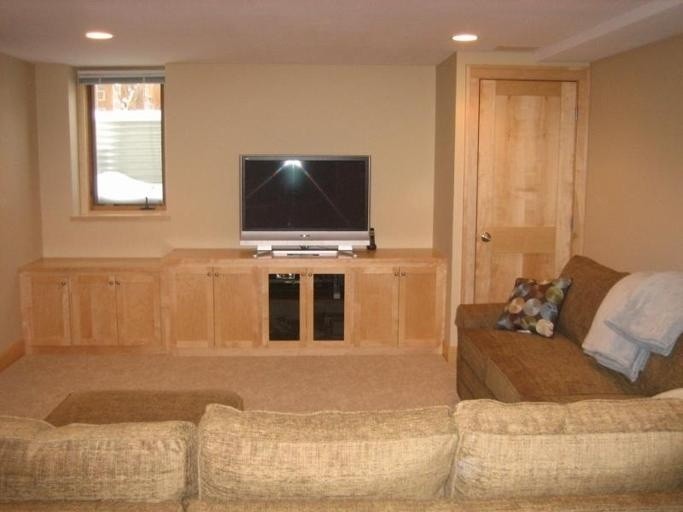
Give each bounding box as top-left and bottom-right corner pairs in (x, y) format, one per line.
(0, 256), (682, 510)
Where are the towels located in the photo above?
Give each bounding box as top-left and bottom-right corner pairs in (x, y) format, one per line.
(578, 268), (682, 381)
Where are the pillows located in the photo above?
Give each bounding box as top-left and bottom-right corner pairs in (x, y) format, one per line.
(493, 276), (575, 340)
(0, 411), (198, 500)
(452, 386), (681, 495)
(194, 400), (459, 492)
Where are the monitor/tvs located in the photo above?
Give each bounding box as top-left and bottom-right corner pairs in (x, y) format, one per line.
(239, 154), (372, 258)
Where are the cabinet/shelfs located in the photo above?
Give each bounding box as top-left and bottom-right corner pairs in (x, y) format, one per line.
(353, 261), (446, 355)
(170, 262), (258, 356)
(260, 260), (352, 355)
(69, 273), (157, 353)
(18, 272), (68, 355)
